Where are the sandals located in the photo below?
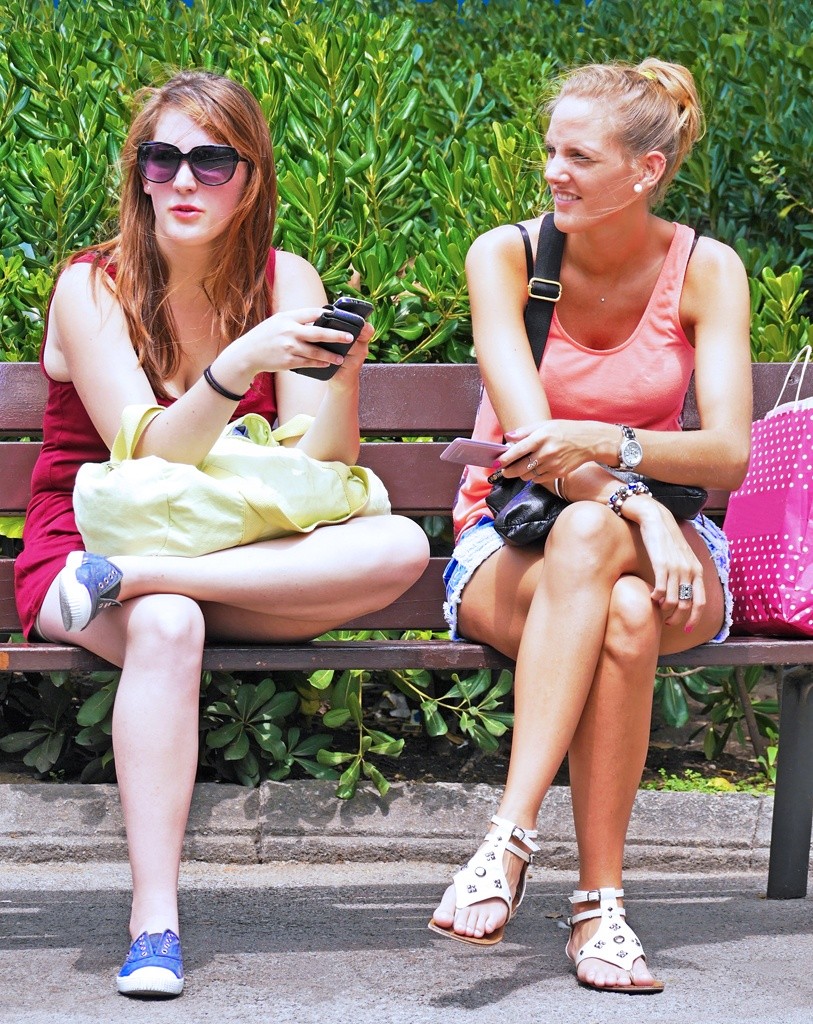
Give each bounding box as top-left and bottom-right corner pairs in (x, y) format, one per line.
(427, 821), (539, 949)
(564, 887), (667, 994)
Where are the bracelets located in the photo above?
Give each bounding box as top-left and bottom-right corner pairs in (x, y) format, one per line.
(606, 482), (652, 517)
(554, 478), (564, 499)
(607, 423), (643, 473)
(204, 365), (245, 401)
(562, 477), (570, 503)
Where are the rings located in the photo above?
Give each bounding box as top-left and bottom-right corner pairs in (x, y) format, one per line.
(532, 469), (541, 478)
(678, 584), (693, 599)
(526, 455), (538, 470)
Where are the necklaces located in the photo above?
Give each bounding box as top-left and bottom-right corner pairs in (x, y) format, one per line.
(601, 298), (605, 302)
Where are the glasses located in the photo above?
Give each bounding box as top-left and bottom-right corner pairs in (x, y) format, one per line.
(137, 140), (252, 186)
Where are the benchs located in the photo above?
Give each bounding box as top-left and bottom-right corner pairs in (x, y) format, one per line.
(1, 359), (812, 899)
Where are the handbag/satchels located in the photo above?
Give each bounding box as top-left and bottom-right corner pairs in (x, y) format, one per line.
(481, 460), (711, 549)
(727, 348), (813, 639)
(69, 402), (391, 561)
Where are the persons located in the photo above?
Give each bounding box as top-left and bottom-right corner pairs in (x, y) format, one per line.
(428, 57), (754, 992)
(13, 70), (430, 995)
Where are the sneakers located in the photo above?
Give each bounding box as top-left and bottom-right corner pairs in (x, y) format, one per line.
(59, 550), (126, 635)
(116, 929), (183, 998)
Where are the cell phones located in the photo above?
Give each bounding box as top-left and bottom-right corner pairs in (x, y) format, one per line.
(439, 437), (532, 470)
(332, 295), (375, 320)
